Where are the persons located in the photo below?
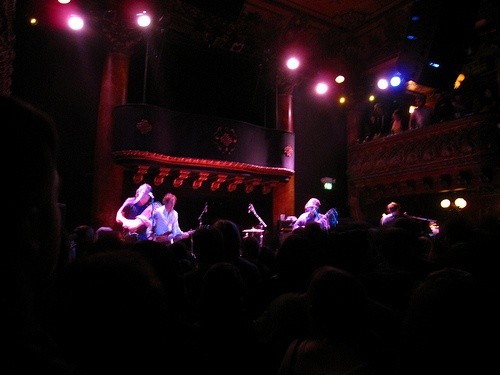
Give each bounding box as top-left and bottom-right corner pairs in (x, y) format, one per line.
(0, 91), (499, 375)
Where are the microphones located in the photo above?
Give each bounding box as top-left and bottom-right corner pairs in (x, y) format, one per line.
(148, 192), (154, 199)
(248, 204), (251, 213)
(205, 202), (208, 212)
